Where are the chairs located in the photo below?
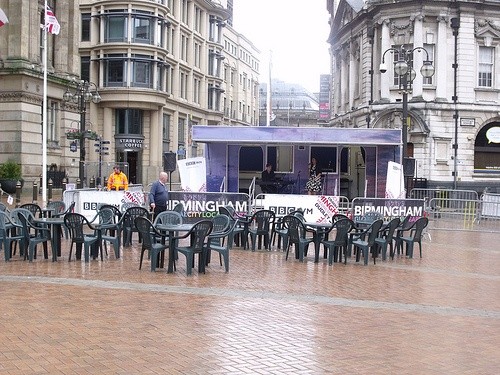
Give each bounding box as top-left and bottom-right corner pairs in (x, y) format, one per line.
(0, 195), (428, 276)
(340, 178), (350, 195)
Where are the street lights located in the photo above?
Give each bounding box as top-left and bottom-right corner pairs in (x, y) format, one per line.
(61, 77), (102, 188)
(377, 46), (435, 185)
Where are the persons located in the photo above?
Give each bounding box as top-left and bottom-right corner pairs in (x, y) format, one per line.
(262, 163), (276, 181)
(107, 166), (128, 190)
(149, 172), (169, 224)
(305, 158), (322, 194)
(126, 163), (137, 184)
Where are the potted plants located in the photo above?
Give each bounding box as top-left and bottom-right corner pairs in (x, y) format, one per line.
(0, 160), (22, 194)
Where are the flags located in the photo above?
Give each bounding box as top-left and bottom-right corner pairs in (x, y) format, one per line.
(0, 8), (9, 27)
(46, 5), (60, 35)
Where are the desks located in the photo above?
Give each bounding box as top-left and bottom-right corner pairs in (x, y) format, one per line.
(245, 214), (285, 245)
(29, 209), (54, 239)
(33, 218), (81, 259)
(110, 212), (154, 244)
(356, 221), (389, 252)
(302, 223), (345, 259)
(157, 224), (211, 260)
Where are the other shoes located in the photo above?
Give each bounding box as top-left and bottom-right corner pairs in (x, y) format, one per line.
(152, 237), (161, 240)
(160, 236), (169, 240)
